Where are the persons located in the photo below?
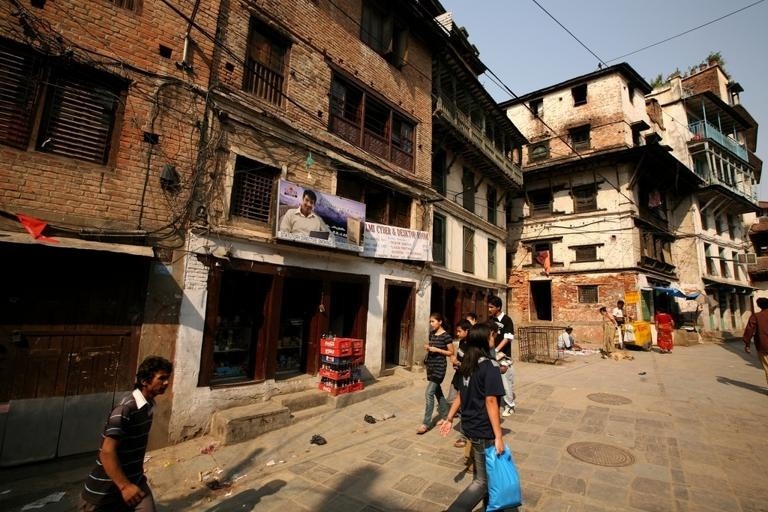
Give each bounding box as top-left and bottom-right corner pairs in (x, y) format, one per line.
(654, 305), (676, 354)
(417, 292), (522, 511)
(611, 300), (628, 350)
(599, 306), (619, 359)
(77, 353), (175, 511)
(742, 297), (768, 388)
(557, 325), (583, 352)
(279, 186), (336, 247)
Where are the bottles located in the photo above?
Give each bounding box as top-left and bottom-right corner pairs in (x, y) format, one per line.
(319, 330), (363, 390)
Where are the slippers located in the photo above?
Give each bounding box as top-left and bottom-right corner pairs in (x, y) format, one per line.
(455, 437), (465, 447)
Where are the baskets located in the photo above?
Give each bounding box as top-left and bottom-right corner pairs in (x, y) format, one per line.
(319, 336), (365, 395)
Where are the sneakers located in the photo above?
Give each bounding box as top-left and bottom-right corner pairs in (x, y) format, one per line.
(501, 407), (515, 417)
(417, 424), (428, 434)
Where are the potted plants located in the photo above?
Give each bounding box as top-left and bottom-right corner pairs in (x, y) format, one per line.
(650, 51), (725, 88)
